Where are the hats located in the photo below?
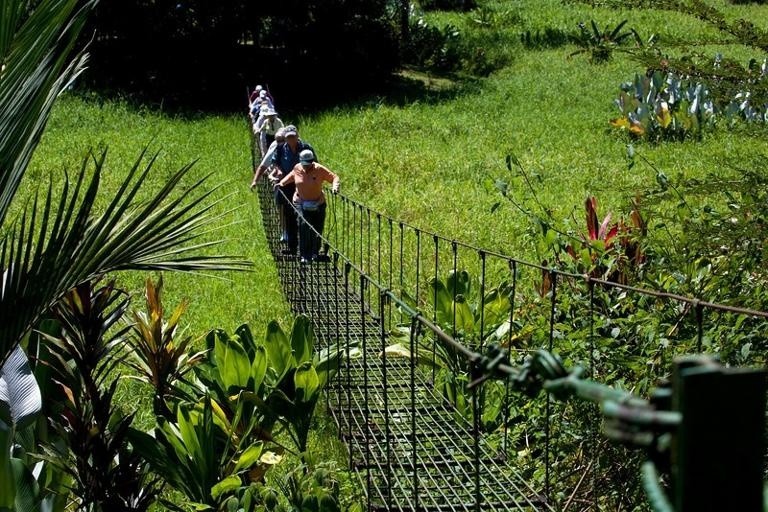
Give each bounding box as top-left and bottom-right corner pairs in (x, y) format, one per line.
(256, 85), (277, 116)
(275, 124), (297, 141)
(299, 149), (314, 167)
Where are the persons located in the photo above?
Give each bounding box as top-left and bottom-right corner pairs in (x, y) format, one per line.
(247, 83), (284, 154)
(273, 124), (318, 255)
(250, 126), (301, 244)
(270, 147), (341, 266)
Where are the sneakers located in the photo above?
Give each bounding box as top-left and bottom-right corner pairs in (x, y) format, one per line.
(300, 253), (317, 264)
